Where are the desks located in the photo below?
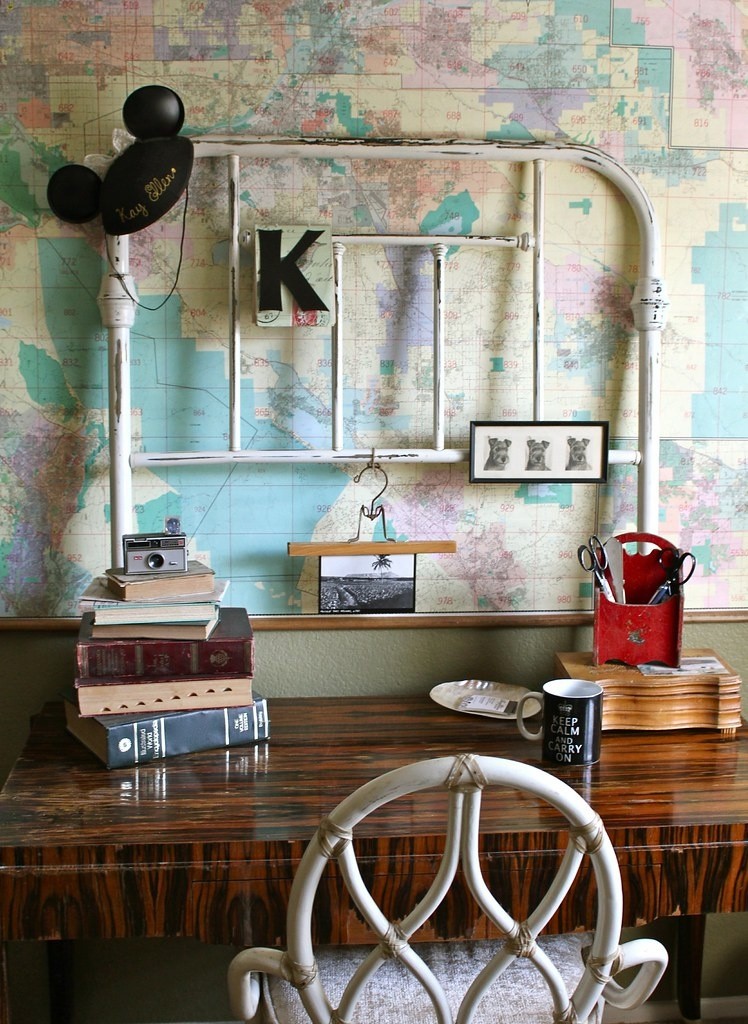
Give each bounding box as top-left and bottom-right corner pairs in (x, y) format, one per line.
(0, 695), (748, 1024)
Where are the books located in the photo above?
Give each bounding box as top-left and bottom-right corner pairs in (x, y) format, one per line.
(73, 673), (255, 718)
(60, 689), (269, 769)
(75, 560), (255, 677)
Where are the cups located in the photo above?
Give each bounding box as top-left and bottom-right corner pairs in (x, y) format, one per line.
(517, 678), (604, 765)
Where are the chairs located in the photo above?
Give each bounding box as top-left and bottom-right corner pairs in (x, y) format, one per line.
(229, 754), (669, 1024)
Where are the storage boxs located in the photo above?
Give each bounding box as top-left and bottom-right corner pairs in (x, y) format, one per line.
(591, 533), (685, 674)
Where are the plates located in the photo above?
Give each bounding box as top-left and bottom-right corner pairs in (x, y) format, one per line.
(429, 680), (541, 719)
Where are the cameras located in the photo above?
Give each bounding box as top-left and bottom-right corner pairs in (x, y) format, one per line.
(122, 533), (189, 576)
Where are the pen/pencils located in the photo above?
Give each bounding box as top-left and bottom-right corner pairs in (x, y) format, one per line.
(646, 580), (672, 605)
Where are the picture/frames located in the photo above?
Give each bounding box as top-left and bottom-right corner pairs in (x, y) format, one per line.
(468, 420), (609, 484)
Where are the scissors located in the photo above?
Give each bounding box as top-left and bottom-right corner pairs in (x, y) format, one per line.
(576, 535), (617, 603)
(658, 547), (697, 595)
